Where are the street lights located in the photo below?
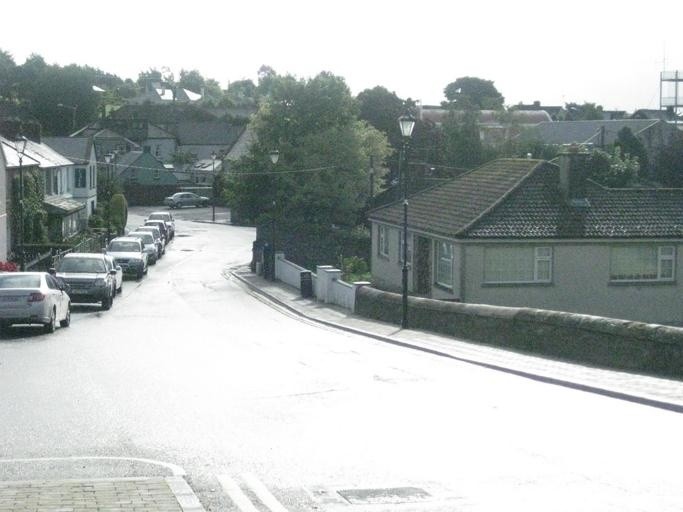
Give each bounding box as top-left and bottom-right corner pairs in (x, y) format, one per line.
(269, 149), (279, 282)
(211, 151), (217, 221)
(396, 112), (416, 327)
(104, 148), (120, 243)
(16, 132), (29, 272)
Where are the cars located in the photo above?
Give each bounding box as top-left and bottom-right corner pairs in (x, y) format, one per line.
(164, 191), (210, 208)
(106, 254), (123, 292)
(1, 270), (72, 333)
(55, 252), (116, 311)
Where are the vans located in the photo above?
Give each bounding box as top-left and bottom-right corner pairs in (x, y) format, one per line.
(128, 211), (176, 265)
(102, 236), (148, 279)
